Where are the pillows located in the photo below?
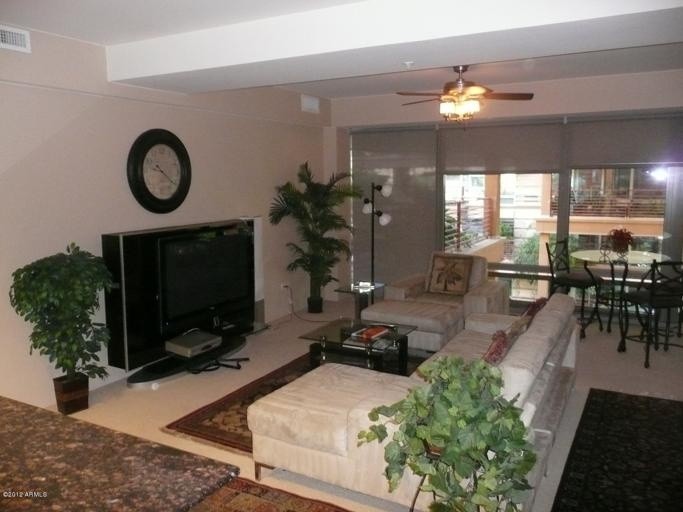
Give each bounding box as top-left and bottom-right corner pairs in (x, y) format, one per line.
(475, 297), (548, 367)
(424, 251), (472, 294)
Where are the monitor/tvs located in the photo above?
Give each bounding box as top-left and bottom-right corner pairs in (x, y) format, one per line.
(101, 219), (254, 388)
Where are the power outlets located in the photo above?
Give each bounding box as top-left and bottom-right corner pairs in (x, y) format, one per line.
(281, 282), (289, 294)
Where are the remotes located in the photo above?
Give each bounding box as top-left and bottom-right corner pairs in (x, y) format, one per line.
(367, 322), (399, 328)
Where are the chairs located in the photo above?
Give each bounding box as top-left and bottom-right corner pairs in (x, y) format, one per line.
(381, 247), (508, 315)
(546, 237), (683, 369)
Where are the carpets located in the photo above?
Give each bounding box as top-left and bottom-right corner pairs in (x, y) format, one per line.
(159, 344), (429, 461)
(188, 474), (342, 511)
(551, 386), (682, 512)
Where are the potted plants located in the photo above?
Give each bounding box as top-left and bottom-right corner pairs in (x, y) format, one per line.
(354, 351), (539, 511)
(6, 241), (120, 418)
(265, 160), (368, 312)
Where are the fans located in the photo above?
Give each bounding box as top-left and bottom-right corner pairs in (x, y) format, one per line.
(392, 65), (535, 113)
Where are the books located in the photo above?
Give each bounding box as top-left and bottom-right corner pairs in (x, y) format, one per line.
(350, 325), (388, 339)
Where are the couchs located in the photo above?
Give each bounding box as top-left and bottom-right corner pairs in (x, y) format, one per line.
(245, 293), (593, 511)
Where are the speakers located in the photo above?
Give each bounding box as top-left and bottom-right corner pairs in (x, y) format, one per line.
(255, 297), (265, 328)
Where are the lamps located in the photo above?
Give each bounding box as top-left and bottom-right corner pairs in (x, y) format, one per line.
(357, 179), (394, 302)
(438, 102), (482, 123)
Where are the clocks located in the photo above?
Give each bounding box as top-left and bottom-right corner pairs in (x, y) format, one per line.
(126, 127), (192, 216)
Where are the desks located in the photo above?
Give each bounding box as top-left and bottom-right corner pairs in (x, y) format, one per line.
(337, 281), (383, 341)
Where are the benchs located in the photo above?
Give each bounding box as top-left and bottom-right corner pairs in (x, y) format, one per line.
(362, 301), (463, 353)
